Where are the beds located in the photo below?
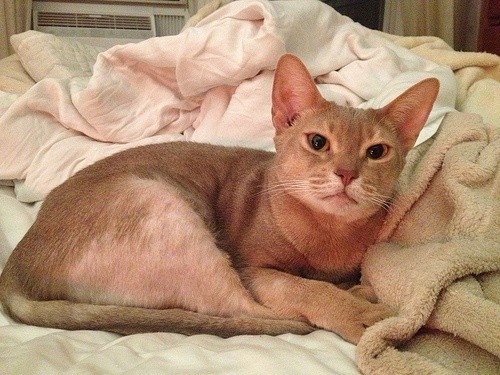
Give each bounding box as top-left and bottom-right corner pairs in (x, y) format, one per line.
(0, 36), (500, 374)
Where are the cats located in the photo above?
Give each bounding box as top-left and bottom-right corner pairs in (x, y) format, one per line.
(0, 52), (440, 345)
